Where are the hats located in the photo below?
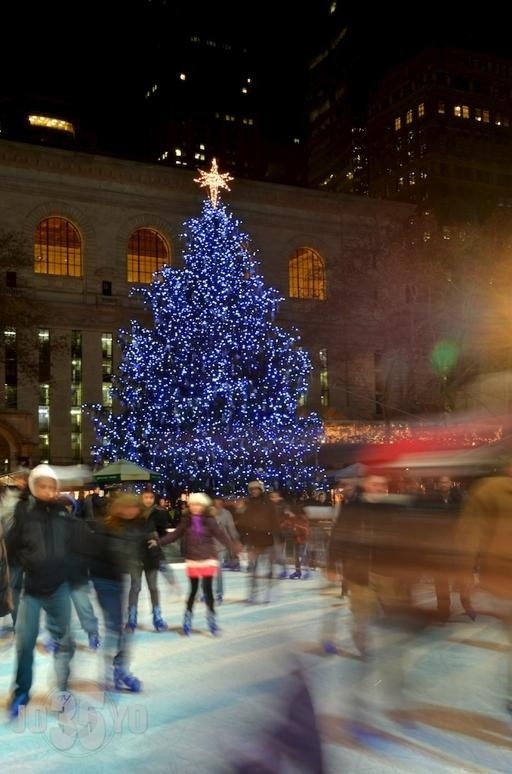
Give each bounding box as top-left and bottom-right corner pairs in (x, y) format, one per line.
(28, 464), (58, 498)
(187, 492), (212, 507)
(248, 481), (265, 493)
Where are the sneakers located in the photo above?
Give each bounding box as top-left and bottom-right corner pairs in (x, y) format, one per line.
(182, 614), (192, 632)
(207, 615), (219, 631)
(437, 605), (478, 621)
(152, 612), (167, 627)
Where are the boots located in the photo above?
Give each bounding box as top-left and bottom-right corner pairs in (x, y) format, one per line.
(113, 668), (139, 688)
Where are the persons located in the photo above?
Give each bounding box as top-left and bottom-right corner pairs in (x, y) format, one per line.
(4, 462), (512, 720)
